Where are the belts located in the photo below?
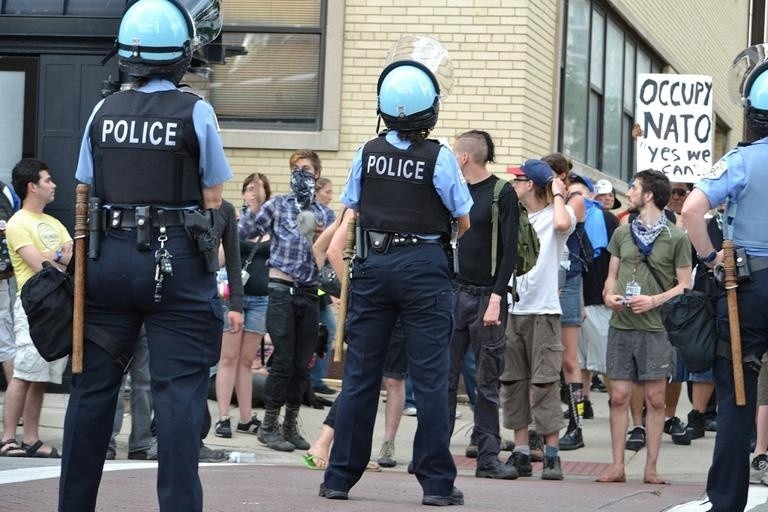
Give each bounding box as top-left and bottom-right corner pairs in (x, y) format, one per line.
(268, 288), (320, 302)
(452, 281), (520, 302)
(749, 258), (767, 274)
(105, 210), (184, 228)
(390, 234), (443, 245)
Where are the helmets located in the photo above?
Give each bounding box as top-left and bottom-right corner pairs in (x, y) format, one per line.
(376, 60), (440, 131)
(740, 61), (767, 129)
(116, 0), (196, 83)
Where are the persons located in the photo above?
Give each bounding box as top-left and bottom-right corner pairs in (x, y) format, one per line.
(316, 58), (473, 506)
(680, 60), (768, 512)
(450, 131), (723, 480)
(58, 0), (233, 512)
(0, 158), (74, 458)
(107, 148), (405, 466)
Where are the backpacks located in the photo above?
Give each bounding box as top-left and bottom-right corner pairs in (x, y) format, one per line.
(0, 182), (15, 281)
(492, 179), (540, 276)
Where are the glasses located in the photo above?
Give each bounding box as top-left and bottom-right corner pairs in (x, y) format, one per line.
(670, 189), (687, 197)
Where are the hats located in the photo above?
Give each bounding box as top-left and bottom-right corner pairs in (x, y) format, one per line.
(507, 158), (622, 210)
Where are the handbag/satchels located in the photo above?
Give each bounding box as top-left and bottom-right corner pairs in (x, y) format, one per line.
(580, 234), (594, 271)
(20, 260), (72, 361)
(659, 289), (718, 374)
(315, 260), (341, 298)
(218, 266), (250, 300)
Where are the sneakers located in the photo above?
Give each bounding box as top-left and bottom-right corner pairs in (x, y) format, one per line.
(423, 487), (464, 505)
(319, 483), (348, 499)
